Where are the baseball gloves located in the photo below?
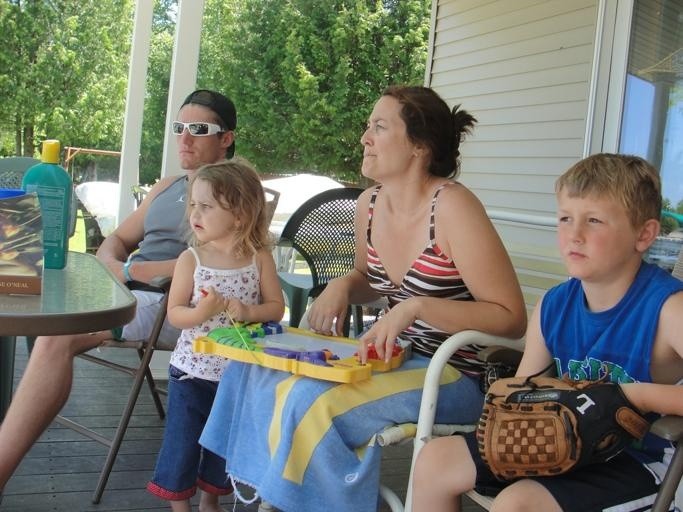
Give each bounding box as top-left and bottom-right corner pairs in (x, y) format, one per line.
(475, 361), (653, 478)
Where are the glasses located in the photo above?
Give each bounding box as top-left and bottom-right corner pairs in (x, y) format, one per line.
(171, 118), (226, 138)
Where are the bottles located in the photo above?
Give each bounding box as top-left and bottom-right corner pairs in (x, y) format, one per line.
(20, 135), (75, 272)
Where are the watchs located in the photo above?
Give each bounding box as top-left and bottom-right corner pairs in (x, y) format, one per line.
(122, 262), (132, 282)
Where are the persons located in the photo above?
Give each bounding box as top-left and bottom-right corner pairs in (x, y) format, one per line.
(0, 89), (239, 494)
(407, 152), (681, 512)
(146, 161), (287, 512)
(194, 80), (531, 512)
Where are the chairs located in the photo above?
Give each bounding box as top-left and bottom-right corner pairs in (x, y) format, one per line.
(55, 188), (281, 504)
(404, 330), (681, 512)
(253, 419), (479, 512)
(76, 181), (180, 348)
(5, 157), (77, 238)
(275, 188), (366, 338)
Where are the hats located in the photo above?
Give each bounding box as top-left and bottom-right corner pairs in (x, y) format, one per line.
(178, 90), (237, 161)
